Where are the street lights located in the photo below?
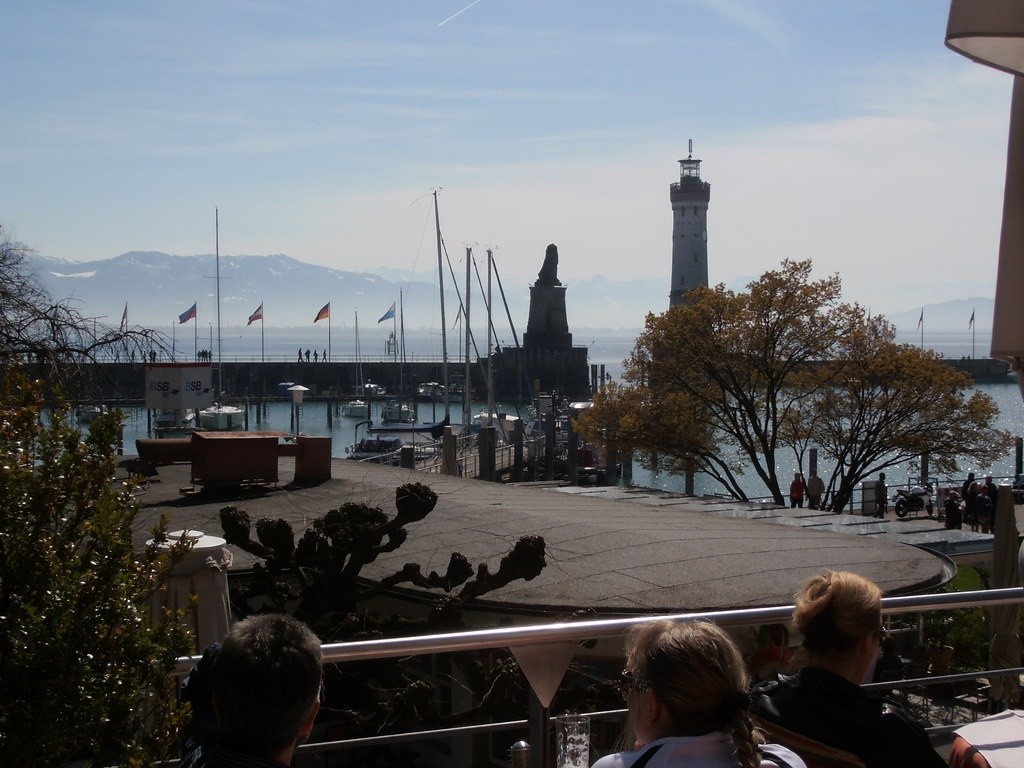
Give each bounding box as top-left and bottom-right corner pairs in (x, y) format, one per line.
(288, 384), (311, 436)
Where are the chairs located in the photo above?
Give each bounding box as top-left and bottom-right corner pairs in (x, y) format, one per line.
(886, 655), (994, 726)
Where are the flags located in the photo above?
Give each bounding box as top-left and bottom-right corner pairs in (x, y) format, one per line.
(314, 302), (330, 323)
(378, 303), (395, 323)
(179, 303), (196, 324)
(248, 304), (263, 325)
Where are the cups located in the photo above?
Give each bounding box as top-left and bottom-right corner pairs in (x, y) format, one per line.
(556, 714), (590, 768)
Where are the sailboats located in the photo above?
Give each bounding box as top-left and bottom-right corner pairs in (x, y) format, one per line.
(342, 311), (368, 418)
(195, 207), (245, 430)
(379, 289), (415, 422)
(344, 189), (539, 466)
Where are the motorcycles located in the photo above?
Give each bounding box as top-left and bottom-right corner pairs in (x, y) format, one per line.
(891, 482), (934, 517)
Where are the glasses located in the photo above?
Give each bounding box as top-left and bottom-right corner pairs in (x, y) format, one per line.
(620, 669), (654, 689)
(876, 626), (888, 639)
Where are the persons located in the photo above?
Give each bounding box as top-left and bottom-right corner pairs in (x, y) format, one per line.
(790, 473), (805, 508)
(298, 348), (327, 362)
(143, 350), (157, 362)
(944, 472), (997, 534)
(873, 473), (886, 519)
(177, 614), (325, 768)
(590, 619), (806, 768)
(750, 569), (948, 768)
(114, 350), (121, 363)
(131, 350), (135, 363)
(1013, 474), (1021, 489)
(807, 469), (825, 510)
(197, 348), (213, 362)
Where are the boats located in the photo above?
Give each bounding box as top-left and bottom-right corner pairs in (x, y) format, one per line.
(77, 403), (108, 422)
(443, 374), (477, 404)
(155, 405), (193, 431)
